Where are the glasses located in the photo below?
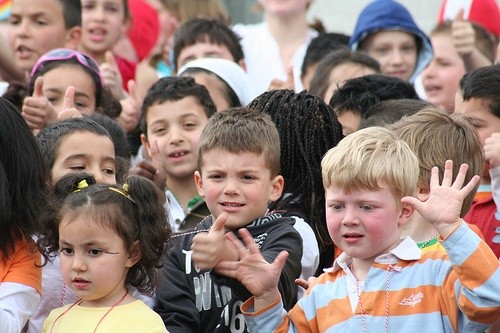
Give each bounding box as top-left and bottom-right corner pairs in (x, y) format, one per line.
(30, 48), (104, 89)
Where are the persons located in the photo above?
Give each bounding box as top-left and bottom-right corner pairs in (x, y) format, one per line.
(0, 0), (500, 333)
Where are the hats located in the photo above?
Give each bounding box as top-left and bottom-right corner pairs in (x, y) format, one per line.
(178, 58), (252, 106)
